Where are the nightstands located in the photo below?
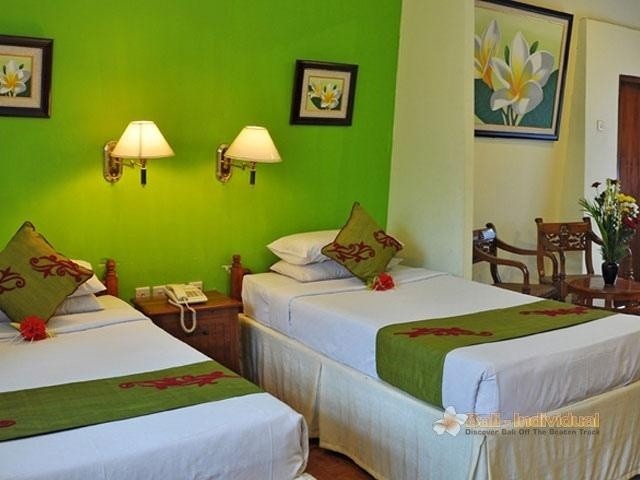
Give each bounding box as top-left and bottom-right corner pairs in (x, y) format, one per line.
(127, 289), (248, 379)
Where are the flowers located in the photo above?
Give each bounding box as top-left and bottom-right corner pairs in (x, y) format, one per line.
(579, 178), (640, 264)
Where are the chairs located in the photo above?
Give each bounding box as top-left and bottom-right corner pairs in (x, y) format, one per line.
(534, 214), (634, 304)
(471, 222), (563, 300)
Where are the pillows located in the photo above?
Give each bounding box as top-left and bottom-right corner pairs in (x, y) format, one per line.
(321, 201), (404, 287)
(263, 227), (405, 267)
(64, 254), (107, 300)
(0, 220), (95, 336)
(266, 259), (406, 283)
(0, 294), (106, 325)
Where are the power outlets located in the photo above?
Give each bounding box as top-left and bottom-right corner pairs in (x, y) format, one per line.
(135, 286), (151, 299)
(152, 284), (167, 298)
(187, 281), (204, 294)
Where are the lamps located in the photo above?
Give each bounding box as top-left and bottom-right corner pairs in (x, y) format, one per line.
(102, 118), (176, 187)
(213, 124), (284, 190)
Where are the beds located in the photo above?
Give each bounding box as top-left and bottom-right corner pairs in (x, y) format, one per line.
(0, 256), (318, 480)
(229, 249), (640, 480)
(560, 269), (640, 316)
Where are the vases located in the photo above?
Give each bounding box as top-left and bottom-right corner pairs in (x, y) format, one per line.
(596, 258), (620, 289)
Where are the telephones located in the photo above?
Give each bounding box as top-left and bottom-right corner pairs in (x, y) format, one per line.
(162, 283), (208, 334)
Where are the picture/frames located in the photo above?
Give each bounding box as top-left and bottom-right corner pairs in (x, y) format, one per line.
(0, 33), (55, 120)
(288, 58), (360, 127)
(470, 0), (575, 143)
